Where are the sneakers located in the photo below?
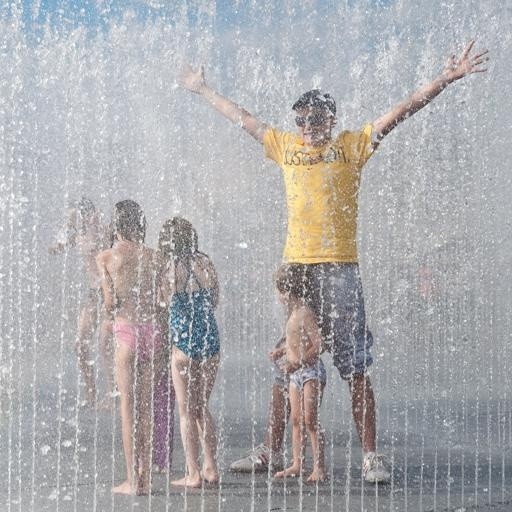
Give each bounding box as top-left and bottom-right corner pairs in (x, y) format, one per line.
(230, 444), (286, 473)
(362, 452), (394, 483)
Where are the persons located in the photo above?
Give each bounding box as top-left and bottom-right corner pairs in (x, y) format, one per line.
(270, 263), (327, 485)
(178, 40), (491, 485)
(48, 198), (117, 411)
(156, 218), (220, 488)
(97, 200), (166, 497)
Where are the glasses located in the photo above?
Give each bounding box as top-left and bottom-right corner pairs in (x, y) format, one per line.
(295, 114), (326, 127)
